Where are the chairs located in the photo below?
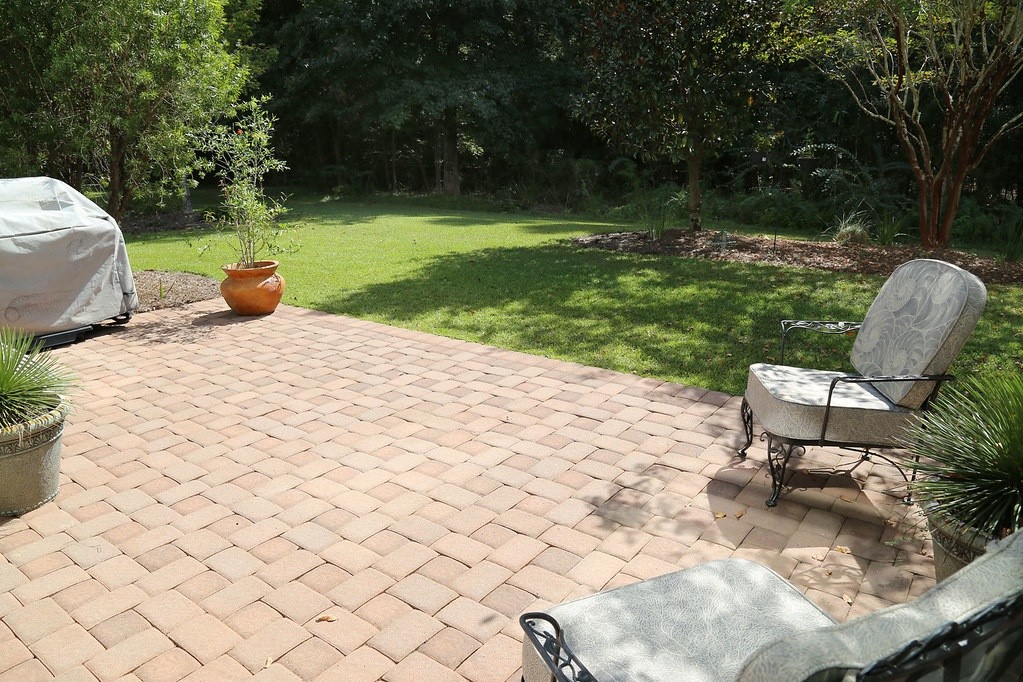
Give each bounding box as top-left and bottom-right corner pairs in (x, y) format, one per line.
(738, 259), (988, 508)
(518, 528), (1023, 682)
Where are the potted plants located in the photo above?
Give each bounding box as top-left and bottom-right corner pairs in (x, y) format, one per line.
(186, 91), (292, 315)
(0, 326), (78, 519)
(886, 362), (1023, 587)
